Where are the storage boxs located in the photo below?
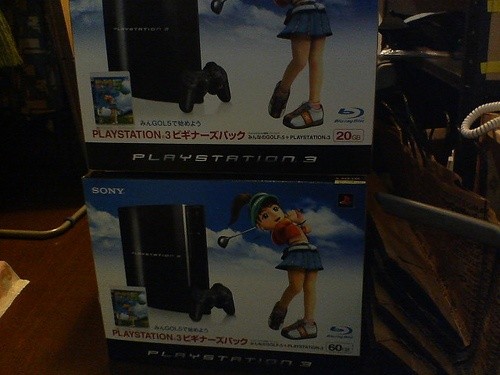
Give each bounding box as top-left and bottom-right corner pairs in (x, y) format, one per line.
(68, 0), (377, 175)
(82, 170), (371, 375)
